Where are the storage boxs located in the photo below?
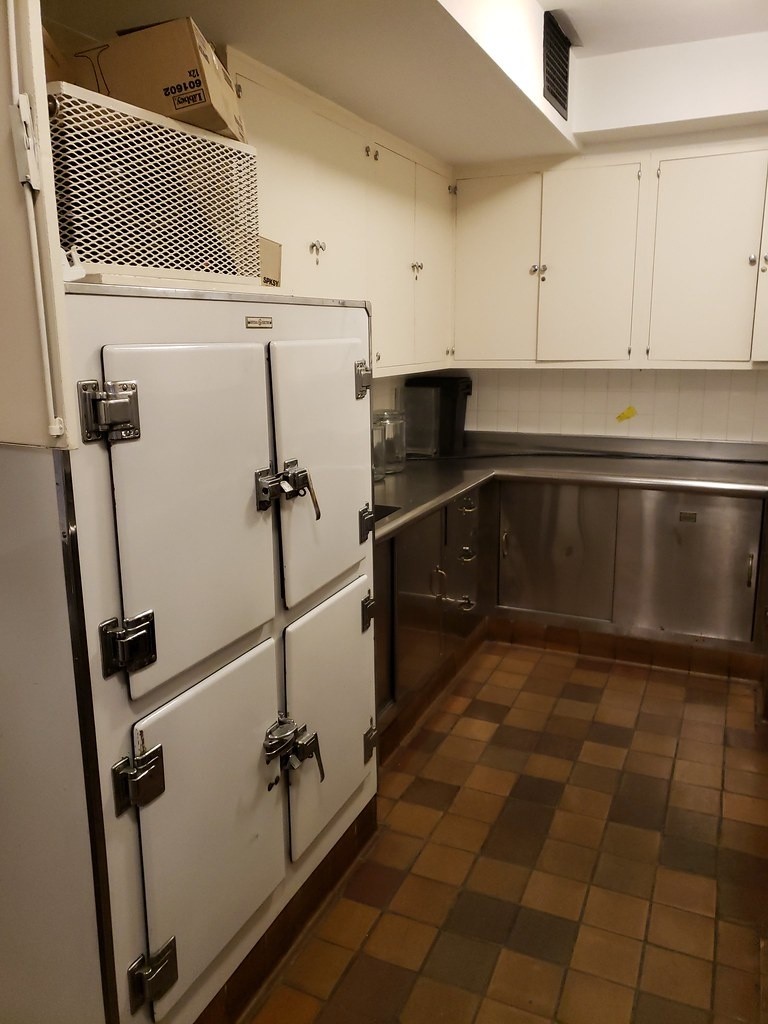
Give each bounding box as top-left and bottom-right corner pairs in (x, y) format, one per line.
(229, 233), (281, 287)
(41, 15), (249, 143)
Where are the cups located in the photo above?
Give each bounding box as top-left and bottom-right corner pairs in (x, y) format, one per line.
(370, 420), (386, 482)
(373, 408), (405, 473)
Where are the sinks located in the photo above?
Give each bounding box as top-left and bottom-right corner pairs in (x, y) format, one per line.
(374, 502), (402, 523)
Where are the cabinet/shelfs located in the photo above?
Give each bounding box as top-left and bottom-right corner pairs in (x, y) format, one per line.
(375, 479), (768, 768)
(1, 280), (397, 1024)
(224, 43), (768, 379)
